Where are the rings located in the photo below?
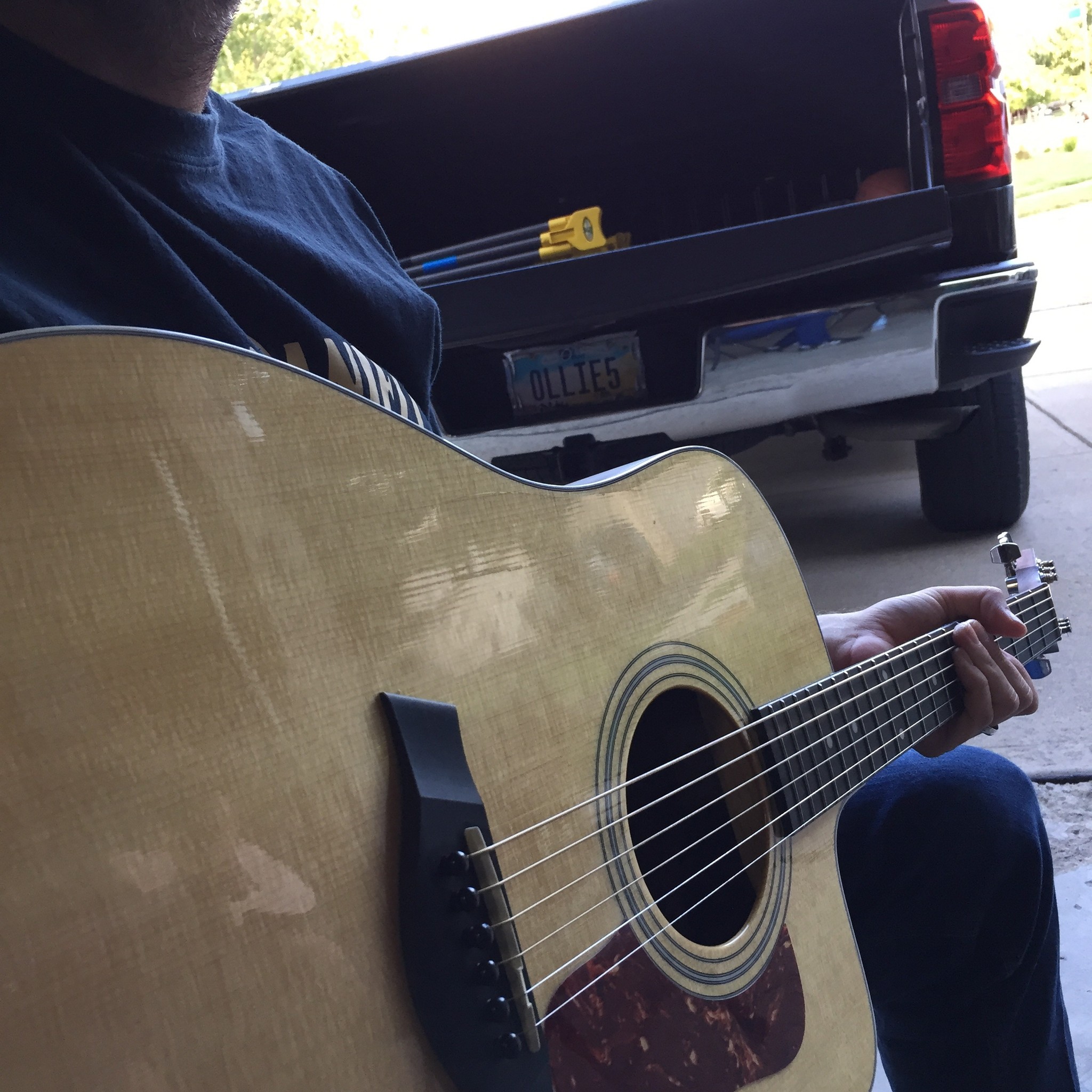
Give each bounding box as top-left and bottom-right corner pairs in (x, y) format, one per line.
(981, 724), (999, 736)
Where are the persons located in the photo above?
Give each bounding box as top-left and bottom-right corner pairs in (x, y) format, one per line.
(0, 1), (1083, 1092)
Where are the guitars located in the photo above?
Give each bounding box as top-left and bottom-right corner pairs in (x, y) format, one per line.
(0, 321), (1073, 1092)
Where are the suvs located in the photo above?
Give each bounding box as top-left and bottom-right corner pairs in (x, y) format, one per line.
(216, 0), (1042, 533)
